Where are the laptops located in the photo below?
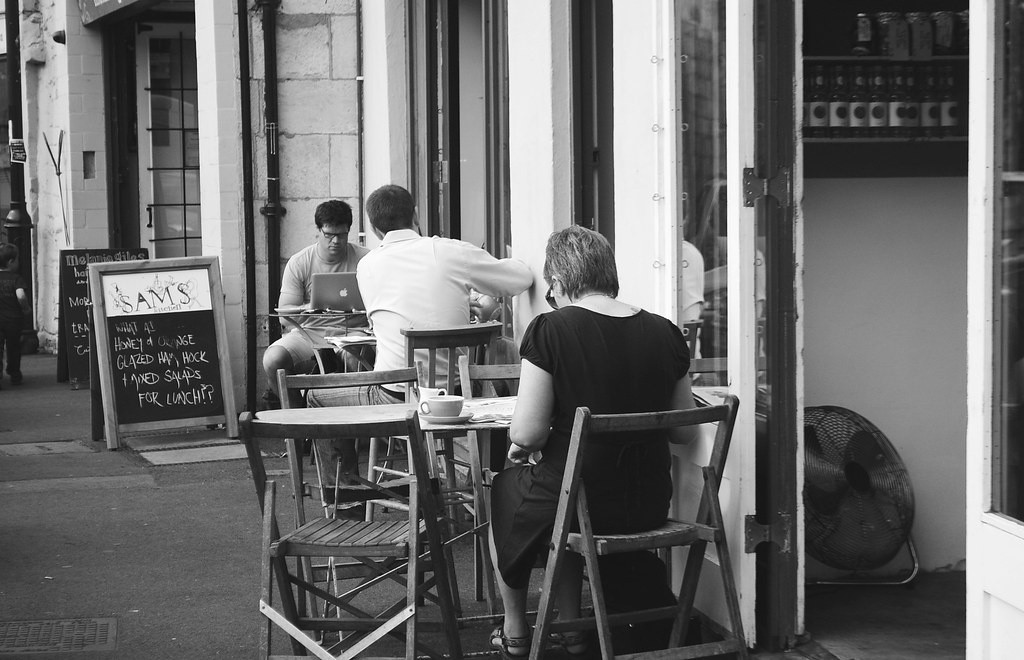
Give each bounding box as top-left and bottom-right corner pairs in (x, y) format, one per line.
(308, 272), (365, 312)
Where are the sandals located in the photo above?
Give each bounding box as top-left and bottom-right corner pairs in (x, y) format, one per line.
(489, 626), (531, 660)
(548, 632), (594, 660)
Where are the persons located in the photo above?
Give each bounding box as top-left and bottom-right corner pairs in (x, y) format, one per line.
(262, 202), (372, 454)
(488, 225), (690, 660)
(678, 239), (706, 387)
(304, 184), (533, 522)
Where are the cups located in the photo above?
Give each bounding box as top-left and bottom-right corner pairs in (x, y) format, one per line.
(477, 294), (501, 321)
(418, 386), (447, 415)
(421, 396), (464, 417)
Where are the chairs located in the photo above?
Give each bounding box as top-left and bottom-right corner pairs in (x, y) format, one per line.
(238, 321), (749, 660)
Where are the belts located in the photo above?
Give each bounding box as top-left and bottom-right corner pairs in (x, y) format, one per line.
(380, 386), (405, 401)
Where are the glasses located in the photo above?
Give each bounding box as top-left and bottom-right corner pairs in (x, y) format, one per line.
(322, 229), (348, 239)
(545, 283), (559, 310)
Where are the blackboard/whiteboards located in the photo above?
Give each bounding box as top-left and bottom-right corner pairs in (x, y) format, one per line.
(84, 254), (239, 453)
(56, 247), (150, 390)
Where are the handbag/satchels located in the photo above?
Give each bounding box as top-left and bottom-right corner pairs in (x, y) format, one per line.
(587, 550), (702, 656)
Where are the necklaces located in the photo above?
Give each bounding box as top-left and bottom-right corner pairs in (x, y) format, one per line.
(574, 292), (610, 302)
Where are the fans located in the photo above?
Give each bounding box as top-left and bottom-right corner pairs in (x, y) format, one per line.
(803, 404), (920, 586)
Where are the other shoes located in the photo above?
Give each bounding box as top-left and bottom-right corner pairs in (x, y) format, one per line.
(301, 438), (312, 456)
(11, 370), (22, 382)
(329, 505), (365, 520)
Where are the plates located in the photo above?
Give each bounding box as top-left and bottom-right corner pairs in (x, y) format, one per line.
(274, 308), (303, 313)
(420, 412), (474, 423)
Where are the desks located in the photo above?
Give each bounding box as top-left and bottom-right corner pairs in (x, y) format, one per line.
(402, 397), (520, 624)
(324, 339), (400, 511)
(269, 313), (374, 375)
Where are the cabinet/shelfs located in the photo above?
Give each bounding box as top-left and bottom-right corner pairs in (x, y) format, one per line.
(801, 0), (969, 176)
